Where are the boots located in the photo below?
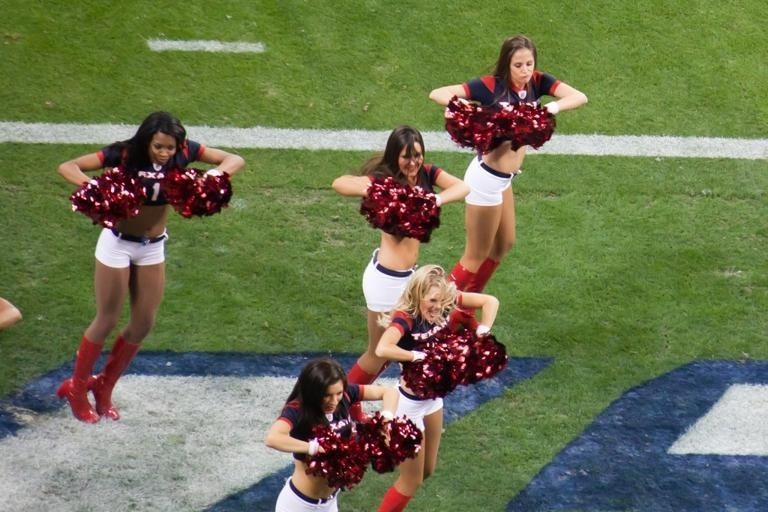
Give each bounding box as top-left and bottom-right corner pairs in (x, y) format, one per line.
(345, 359), (393, 425)
(445, 256), (500, 331)
(57, 335), (102, 423)
(90, 333), (142, 421)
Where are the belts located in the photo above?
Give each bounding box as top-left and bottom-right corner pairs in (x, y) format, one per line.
(399, 386), (429, 401)
(110, 228), (165, 244)
(290, 478), (340, 505)
(373, 248), (417, 277)
(477, 151), (517, 178)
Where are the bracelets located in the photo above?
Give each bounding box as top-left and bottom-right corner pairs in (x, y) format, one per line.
(544, 102), (559, 116)
(88, 179), (99, 190)
(307, 438), (319, 456)
(381, 410), (393, 420)
(434, 195), (442, 207)
(203, 168), (221, 180)
(411, 350), (426, 362)
(477, 325), (490, 334)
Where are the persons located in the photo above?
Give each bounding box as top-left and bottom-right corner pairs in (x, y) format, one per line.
(56, 111), (246, 423)
(265, 358), (401, 512)
(332, 124), (470, 425)
(0, 297), (24, 331)
(375, 264), (501, 512)
(429, 34), (588, 315)
